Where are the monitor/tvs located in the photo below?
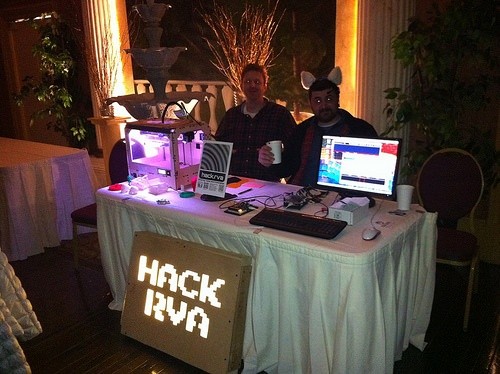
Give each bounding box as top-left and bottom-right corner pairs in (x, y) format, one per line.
(317, 134), (403, 208)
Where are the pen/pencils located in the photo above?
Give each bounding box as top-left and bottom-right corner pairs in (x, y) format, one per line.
(238, 188), (252, 195)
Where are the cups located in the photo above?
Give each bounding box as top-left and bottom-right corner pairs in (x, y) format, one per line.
(266, 140), (282, 164)
(396, 185), (414, 210)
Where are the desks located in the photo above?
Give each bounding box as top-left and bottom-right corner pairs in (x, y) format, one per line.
(95, 174), (438, 374)
(0, 136), (101, 262)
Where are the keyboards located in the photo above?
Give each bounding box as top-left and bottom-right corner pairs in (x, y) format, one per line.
(249, 208), (347, 240)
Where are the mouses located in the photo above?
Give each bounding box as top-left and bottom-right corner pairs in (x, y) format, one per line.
(362, 228), (381, 241)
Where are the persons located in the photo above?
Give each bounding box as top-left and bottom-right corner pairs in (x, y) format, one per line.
(258, 67), (380, 196)
(215, 64), (297, 181)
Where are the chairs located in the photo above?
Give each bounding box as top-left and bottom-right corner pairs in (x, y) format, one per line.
(416, 147), (484, 332)
(72, 138), (146, 272)
